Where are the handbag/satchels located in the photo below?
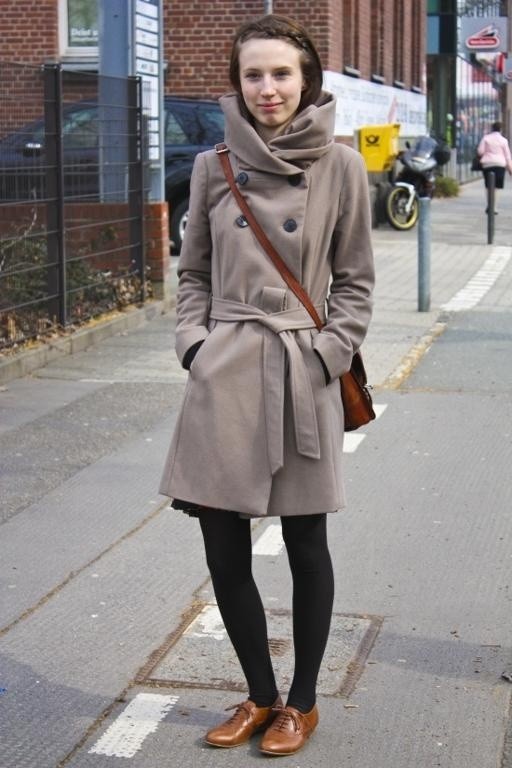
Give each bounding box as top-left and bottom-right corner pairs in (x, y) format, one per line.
(472, 156), (482, 170)
(339, 348), (376, 431)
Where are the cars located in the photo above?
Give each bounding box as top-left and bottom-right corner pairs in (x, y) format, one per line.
(0, 96), (225, 255)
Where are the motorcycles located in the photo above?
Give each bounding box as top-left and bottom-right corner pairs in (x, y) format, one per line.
(386, 136), (451, 231)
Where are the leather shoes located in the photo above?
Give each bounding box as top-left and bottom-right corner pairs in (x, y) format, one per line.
(204, 697), (318, 756)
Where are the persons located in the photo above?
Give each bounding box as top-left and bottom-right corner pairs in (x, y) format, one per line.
(158, 19), (377, 758)
(475, 122), (512, 214)
